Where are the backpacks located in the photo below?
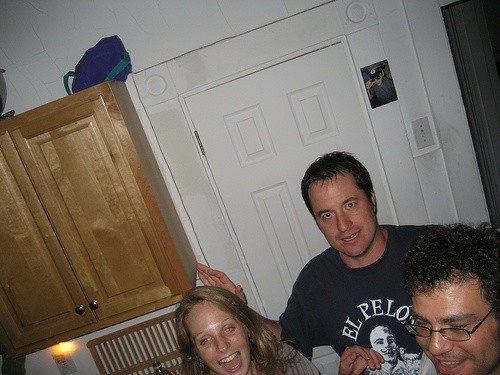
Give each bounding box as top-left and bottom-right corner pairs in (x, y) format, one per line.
(63, 35), (132, 95)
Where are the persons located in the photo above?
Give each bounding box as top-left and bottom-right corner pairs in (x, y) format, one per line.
(365, 62), (399, 105)
(405, 219), (500, 375)
(195, 152), (446, 375)
(175, 286), (387, 375)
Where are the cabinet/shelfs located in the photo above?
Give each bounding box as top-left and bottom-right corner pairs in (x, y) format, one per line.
(0, 80), (198, 360)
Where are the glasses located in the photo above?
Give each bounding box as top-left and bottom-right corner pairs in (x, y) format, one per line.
(404, 307), (492, 341)
(369, 76), (381, 83)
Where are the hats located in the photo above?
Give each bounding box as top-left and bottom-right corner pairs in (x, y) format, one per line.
(365, 64), (386, 74)
